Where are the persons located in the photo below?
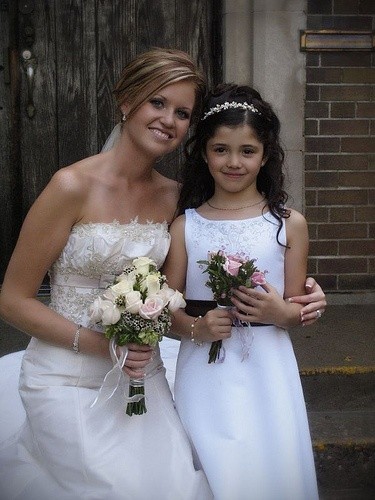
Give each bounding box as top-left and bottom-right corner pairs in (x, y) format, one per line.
(1, 47), (327, 500)
(160, 81), (321, 500)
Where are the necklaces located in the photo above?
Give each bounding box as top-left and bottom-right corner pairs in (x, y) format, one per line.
(206, 196), (266, 211)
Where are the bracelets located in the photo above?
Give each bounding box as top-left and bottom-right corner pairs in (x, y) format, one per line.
(188, 313), (206, 350)
(72, 324), (87, 353)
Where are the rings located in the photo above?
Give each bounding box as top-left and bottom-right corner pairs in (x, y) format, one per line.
(317, 310), (321, 318)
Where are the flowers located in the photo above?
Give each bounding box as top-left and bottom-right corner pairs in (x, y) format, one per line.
(90, 256), (187, 416)
(197, 249), (268, 363)
